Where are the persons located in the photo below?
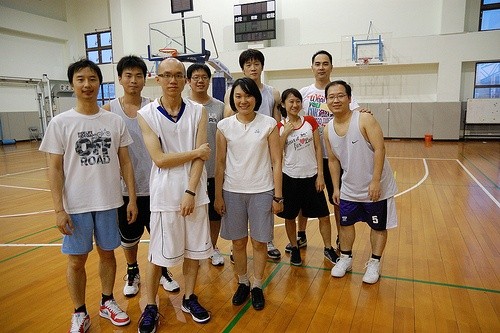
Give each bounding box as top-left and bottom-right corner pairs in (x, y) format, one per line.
(278, 88), (339, 266)
(102, 55), (180, 298)
(213, 77), (284, 310)
(223, 49), (281, 265)
(38, 58), (139, 333)
(136, 58), (215, 333)
(183, 63), (224, 266)
(285, 49), (373, 252)
(323, 80), (398, 284)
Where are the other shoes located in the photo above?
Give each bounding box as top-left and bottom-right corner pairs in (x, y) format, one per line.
(232, 281), (251, 307)
(251, 287), (265, 310)
(290, 246), (303, 266)
(323, 247), (340, 265)
(336, 235), (341, 251)
(285, 231), (307, 253)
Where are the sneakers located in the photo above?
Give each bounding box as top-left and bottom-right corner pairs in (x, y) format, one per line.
(266, 241), (281, 259)
(181, 293), (210, 323)
(123, 267), (141, 297)
(98, 297), (131, 326)
(208, 248), (224, 266)
(361, 258), (382, 284)
(331, 251), (353, 279)
(229, 250), (235, 264)
(138, 303), (165, 333)
(69, 312), (92, 333)
(159, 269), (180, 293)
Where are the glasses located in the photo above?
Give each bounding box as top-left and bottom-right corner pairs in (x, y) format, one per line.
(191, 76), (210, 81)
(157, 73), (186, 80)
(326, 93), (347, 100)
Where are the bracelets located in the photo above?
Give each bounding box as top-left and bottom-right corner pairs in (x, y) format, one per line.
(273, 196), (284, 204)
(185, 189), (196, 197)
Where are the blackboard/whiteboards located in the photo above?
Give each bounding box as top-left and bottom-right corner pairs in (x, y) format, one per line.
(466, 98), (500, 123)
